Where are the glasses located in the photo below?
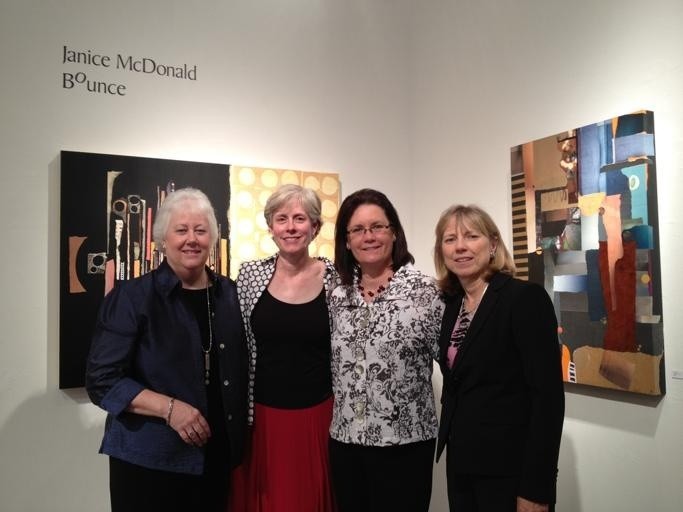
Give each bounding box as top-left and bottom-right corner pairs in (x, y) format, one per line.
(347, 224), (394, 236)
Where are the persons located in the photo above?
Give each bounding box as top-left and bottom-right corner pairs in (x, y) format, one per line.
(432, 205), (565, 512)
(83, 186), (249, 511)
(320, 189), (447, 512)
(227, 183), (345, 512)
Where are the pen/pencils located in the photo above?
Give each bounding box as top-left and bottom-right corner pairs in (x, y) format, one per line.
(205, 350), (210, 385)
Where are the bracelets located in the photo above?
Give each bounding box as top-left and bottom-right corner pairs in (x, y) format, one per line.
(166, 397), (174, 427)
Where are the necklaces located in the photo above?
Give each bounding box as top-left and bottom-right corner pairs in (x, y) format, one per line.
(200, 271), (212, 387)
(357, 267), (392, 297)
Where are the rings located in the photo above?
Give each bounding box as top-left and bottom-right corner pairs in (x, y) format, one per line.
(188, 432), (194, 437)
